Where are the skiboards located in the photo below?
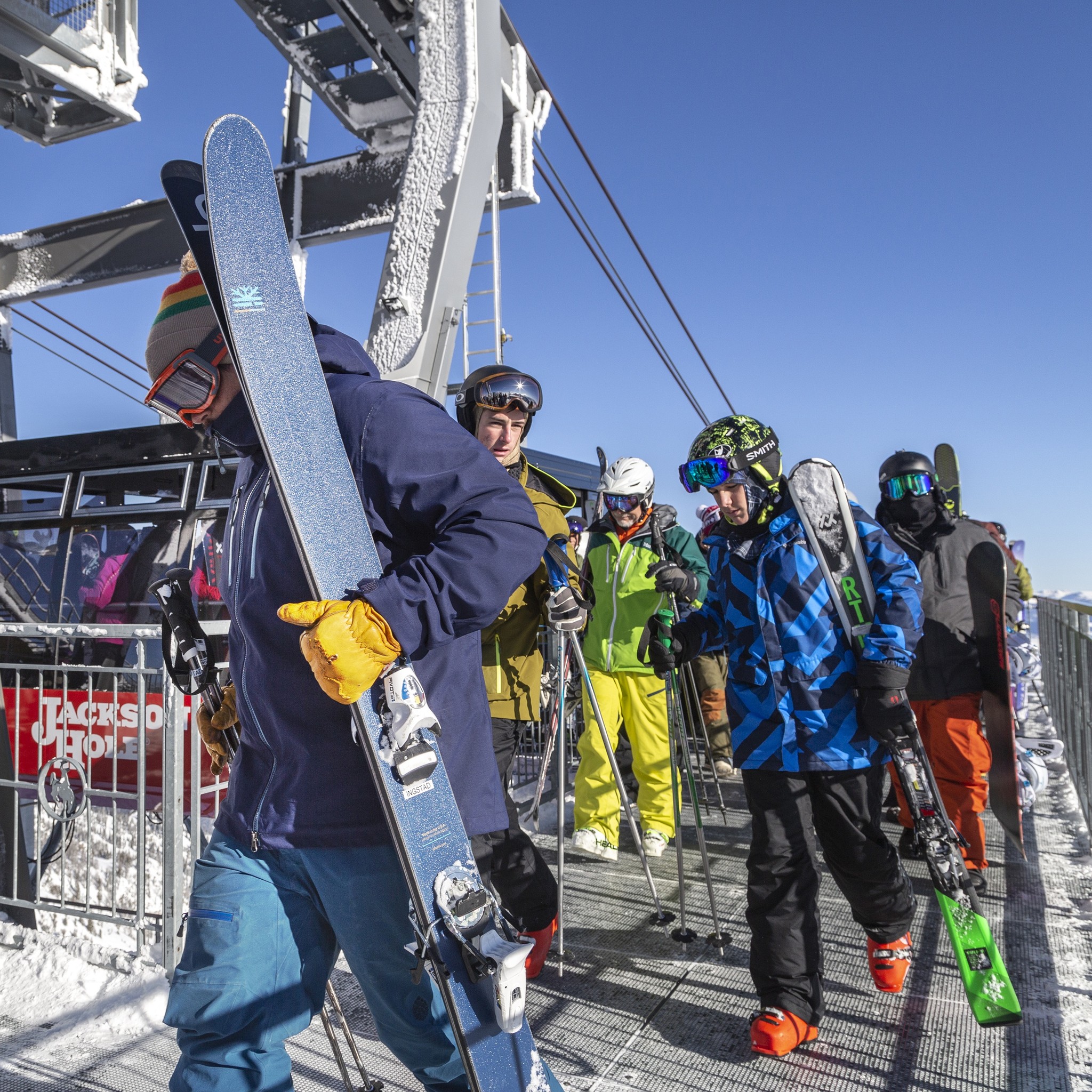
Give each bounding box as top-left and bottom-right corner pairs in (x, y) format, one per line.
(523, 445), (609, 832)
(781, 455), (1022, 1027)
(154, 112), (556, 1092)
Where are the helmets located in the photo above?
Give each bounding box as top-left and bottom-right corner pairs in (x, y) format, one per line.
(456, 365), (533, 444)
(564, 415), (782, 560)
(991, 522), (1006, 535)
(879, 450), (939, 493)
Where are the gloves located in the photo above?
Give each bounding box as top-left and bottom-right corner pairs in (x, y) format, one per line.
(545, 584), (587, 631)
(198, 683), (242, 776)
(854, 659), (918, 756)
(647, 623), (701, 679)
(277, 600), (402, 705)
(644, 560), (700, 603)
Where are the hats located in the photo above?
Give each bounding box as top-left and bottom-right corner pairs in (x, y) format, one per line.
(145, 250), (233, 384)
(473, 406), (528, 466)
(696, 504), (721, 531)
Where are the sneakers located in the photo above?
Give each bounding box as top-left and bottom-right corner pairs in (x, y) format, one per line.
(750, 1005), (819, 1056)
(572, 757), (733, 861)
(516, 911), (560, 980)
(899, 827), (925, 860)
(867, 930), (912, 992)
(968, 869), (986, 895)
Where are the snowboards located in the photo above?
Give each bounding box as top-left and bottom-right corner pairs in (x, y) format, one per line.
(931, 443), (963, 517)
(959, 538), (1025, 857)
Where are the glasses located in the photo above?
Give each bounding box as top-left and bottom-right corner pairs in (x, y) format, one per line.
(474, 372), (543, 412)
(568, 521), (583, 534)
(603, 492), (640, 513)
(679, 454), (734, 493)
(883, 474), (931, 501)
(145, 349), (220, 429)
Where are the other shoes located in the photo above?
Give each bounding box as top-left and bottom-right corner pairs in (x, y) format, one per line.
(887, 806), (901, 823)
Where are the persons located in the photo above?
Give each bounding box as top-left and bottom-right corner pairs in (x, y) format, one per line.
(687, 505), (733, 776)
(570, 457), (710, 861)
(0, 519), (227, 687)
(457, 364), (590, 980)
(567, 516), (589, 570)
(649, 416), (922, 1056)
(989, 521), (1034, 630)
(145, 269), (544, 1091)
(873, 453), (1026, 894)
